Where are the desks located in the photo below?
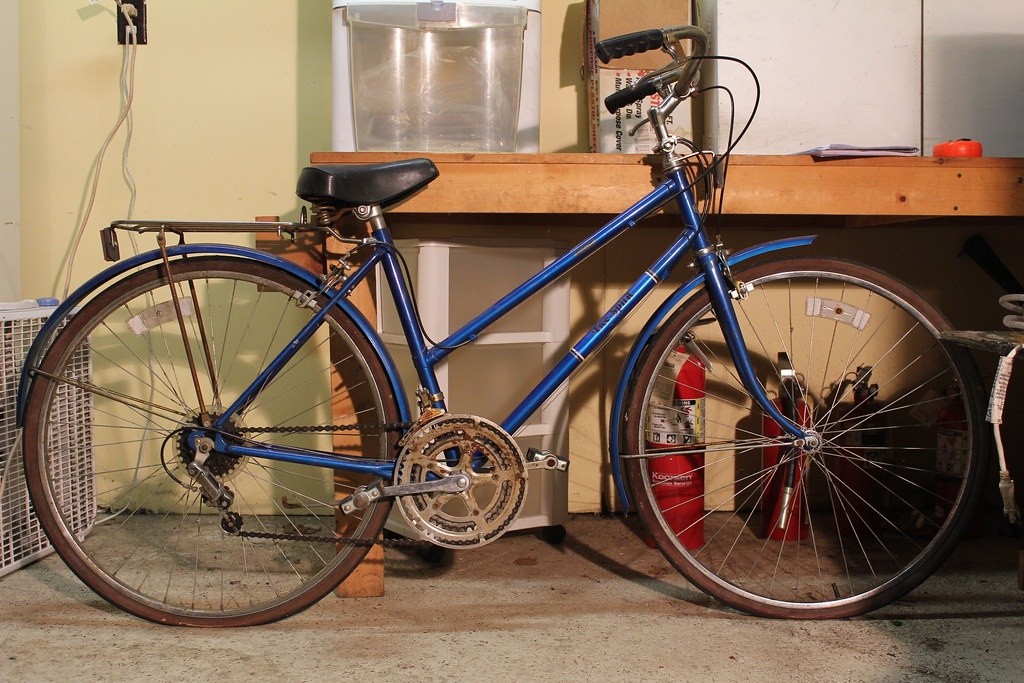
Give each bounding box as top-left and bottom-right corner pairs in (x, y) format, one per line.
(311, 149), (1022, 601)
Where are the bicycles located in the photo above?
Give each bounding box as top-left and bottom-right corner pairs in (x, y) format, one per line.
(13, 21), (997, 630)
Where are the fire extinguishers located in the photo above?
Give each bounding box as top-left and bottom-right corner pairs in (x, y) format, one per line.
(644, 318), (717, 550)
(763, 352), (812, 541)
(934, 371), (985, 541)
(834, 365), (887, 539)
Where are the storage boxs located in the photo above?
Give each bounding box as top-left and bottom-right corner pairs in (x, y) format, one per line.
(335, 3), (534, 153)
(583, 2), (705, 155)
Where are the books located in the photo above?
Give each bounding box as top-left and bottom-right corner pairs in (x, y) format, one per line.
(786, 144), (923, 159)
